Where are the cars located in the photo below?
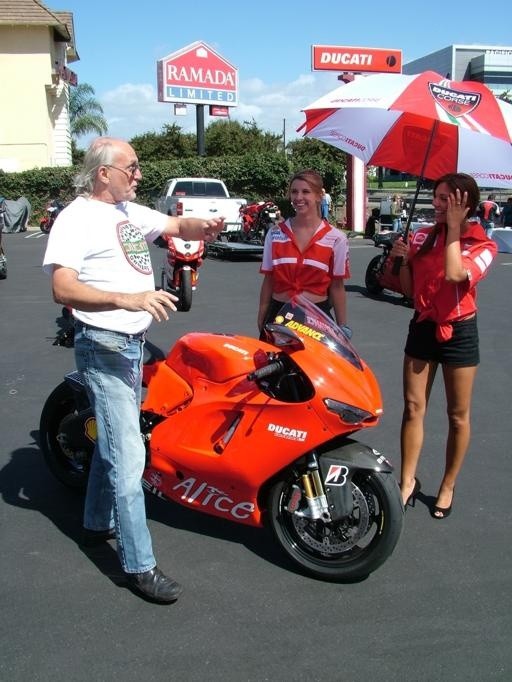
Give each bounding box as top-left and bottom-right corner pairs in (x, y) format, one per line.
(257, 201), (281, 220)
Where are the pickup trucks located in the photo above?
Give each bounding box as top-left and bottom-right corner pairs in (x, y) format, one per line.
(152, 177), (247, 241)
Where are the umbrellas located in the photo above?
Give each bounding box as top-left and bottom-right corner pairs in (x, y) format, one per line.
(295, 69), (512, 276)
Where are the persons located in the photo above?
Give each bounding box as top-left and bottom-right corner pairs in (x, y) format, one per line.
(478, 192), (501, 238)
(320, 188), (332, 222)
(257, 170), (351, 346)
(42, 136), (225, 606)
(387, 191), (408, 233)
(389, 172), (499, 520)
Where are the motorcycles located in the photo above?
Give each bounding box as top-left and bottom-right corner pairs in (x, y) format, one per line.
(39, 200), (64, 234)
(365, 230), (416, 306)
(157, 235), (206, 313)
(36, 293), (406, 584)
(1, 208), (9, 281)
(239, 201), (280, 244)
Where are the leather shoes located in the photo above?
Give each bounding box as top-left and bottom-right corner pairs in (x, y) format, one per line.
(83, 528), (116, 543)
(125, 563), (183, 602)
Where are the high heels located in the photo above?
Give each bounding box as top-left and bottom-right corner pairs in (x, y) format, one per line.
(404, 477), (421, 512)
(433, 486), (455, 520)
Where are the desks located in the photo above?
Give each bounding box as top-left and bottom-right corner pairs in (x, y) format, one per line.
(410, 222), (511, 254)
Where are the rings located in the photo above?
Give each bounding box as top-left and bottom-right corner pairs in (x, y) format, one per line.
(457, 202), (461, 206)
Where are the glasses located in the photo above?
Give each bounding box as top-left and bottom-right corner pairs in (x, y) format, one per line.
(106, 163), (143, 177)
